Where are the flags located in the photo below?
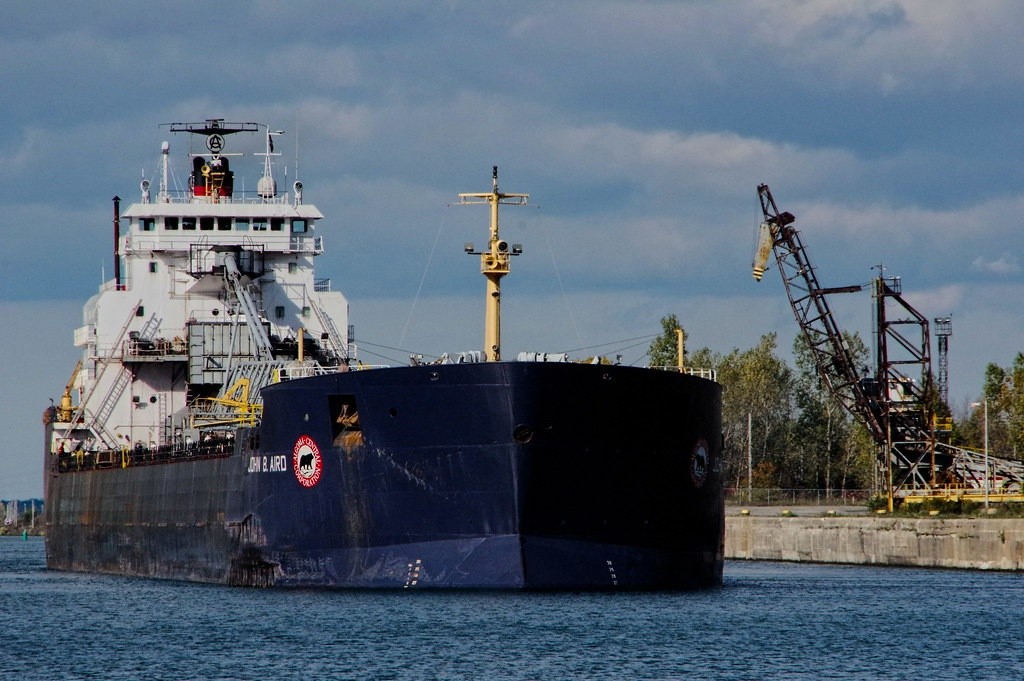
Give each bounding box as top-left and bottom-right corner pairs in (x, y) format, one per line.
(268, 130), (274, 152)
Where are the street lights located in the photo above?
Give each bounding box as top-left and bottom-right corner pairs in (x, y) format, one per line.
(971, 401), (988, 510)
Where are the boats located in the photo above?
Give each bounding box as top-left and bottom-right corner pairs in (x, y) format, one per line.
(42, 118), (724, 595)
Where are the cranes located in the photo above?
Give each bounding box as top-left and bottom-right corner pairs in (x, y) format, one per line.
(753, 183), (952, 485)
